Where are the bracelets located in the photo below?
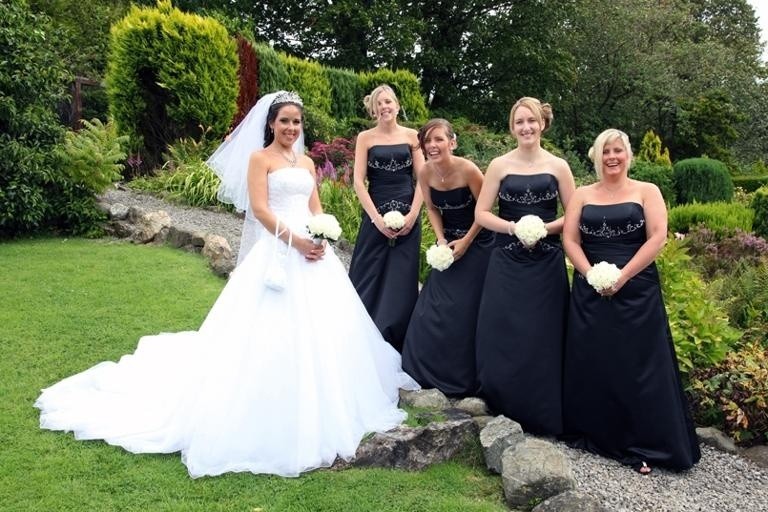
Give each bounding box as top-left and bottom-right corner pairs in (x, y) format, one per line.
(436, 238), (448, 244)
(508, 220), (516, 237)
(371, 214), (382, 224)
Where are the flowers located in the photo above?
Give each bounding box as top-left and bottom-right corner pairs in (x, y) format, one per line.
(426, 245), (454, 272)
(586, 261), (622, 293)
(383, 209), (407, 231)
(513, 214), (548, 246)
(306, 213), (342, 241)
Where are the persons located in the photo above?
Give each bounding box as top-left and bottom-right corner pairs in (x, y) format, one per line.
(352, 84), (426, 355)
(401, 119), (486, 395)
(561, 127), (703, 474)
(473, 96), (576, 435)
(199, 91), (400, 476)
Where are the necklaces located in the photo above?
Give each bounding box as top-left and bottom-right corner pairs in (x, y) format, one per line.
(432, 163), (452, 183)
